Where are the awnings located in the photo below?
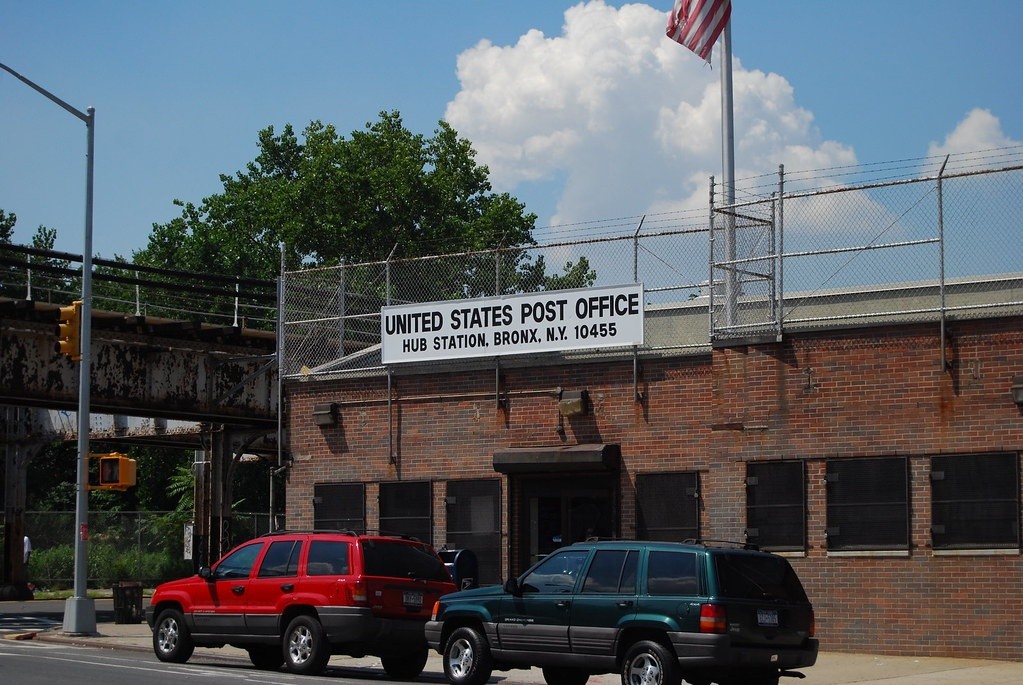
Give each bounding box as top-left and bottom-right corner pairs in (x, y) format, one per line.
(493, 444), (622, 473)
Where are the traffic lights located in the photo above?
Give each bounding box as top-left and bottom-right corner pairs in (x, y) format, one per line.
(99, 457), (136, 487)
(54, 301), (81, 356)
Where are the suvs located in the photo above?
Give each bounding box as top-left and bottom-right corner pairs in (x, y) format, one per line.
(145, 530), (455, 678)
(424, 536), (820, 685)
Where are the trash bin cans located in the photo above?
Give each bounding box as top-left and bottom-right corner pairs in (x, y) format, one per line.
(437, 545), (480, 591)
(111, 584), (144, 625)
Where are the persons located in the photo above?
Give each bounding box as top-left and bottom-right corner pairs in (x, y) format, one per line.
(23, 536), (31, 584)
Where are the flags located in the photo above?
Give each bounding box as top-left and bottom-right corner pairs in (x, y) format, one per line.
(665, 0), (731, 64)
